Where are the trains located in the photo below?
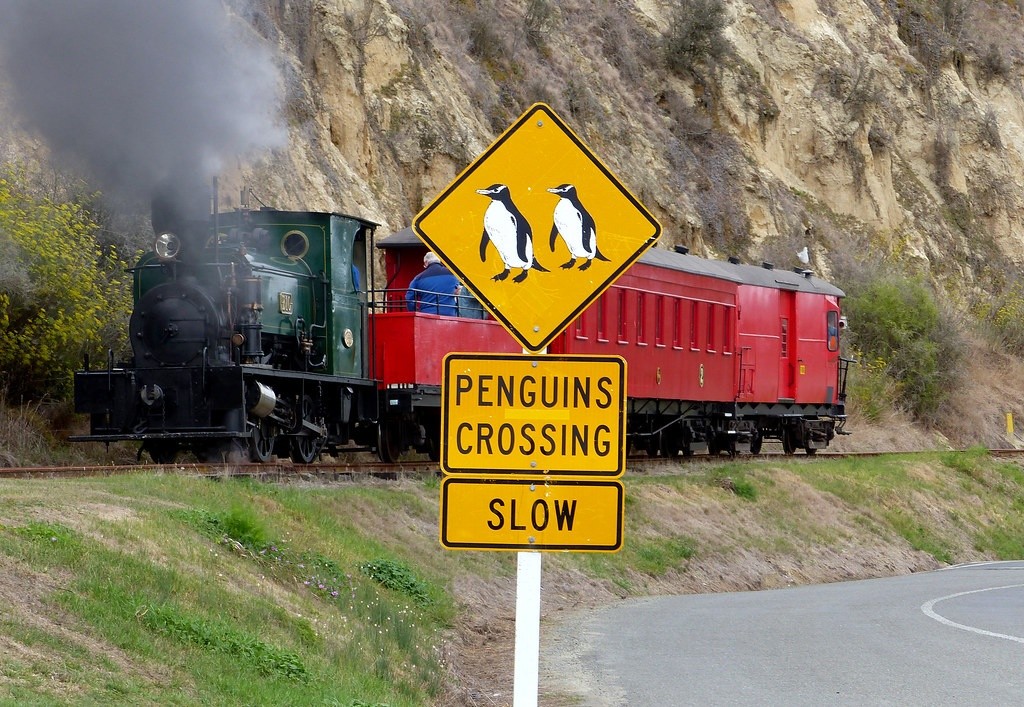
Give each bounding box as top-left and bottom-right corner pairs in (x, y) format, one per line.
(64, 160), (860, 456)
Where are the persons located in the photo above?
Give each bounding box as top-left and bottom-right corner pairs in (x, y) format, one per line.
(406, 252), (459, 317)
